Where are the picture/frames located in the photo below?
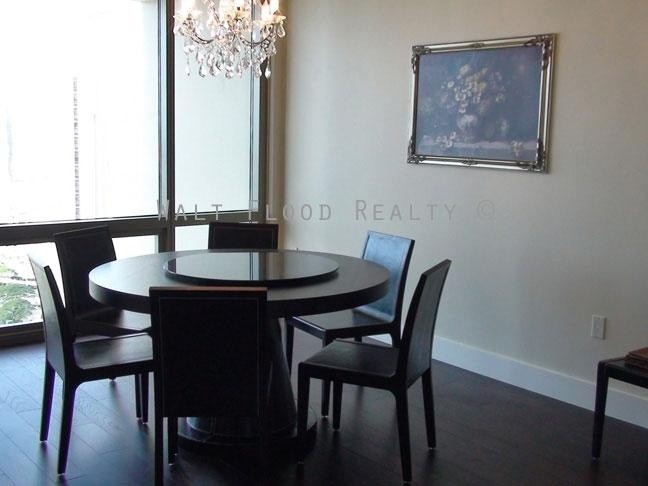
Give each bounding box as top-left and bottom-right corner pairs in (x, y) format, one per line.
(405, 32), (557, 174)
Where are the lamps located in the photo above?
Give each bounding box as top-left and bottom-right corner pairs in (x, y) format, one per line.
(172, 0), (288, 81)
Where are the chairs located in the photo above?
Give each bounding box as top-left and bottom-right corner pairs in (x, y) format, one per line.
(26, 219), (452, 485)
